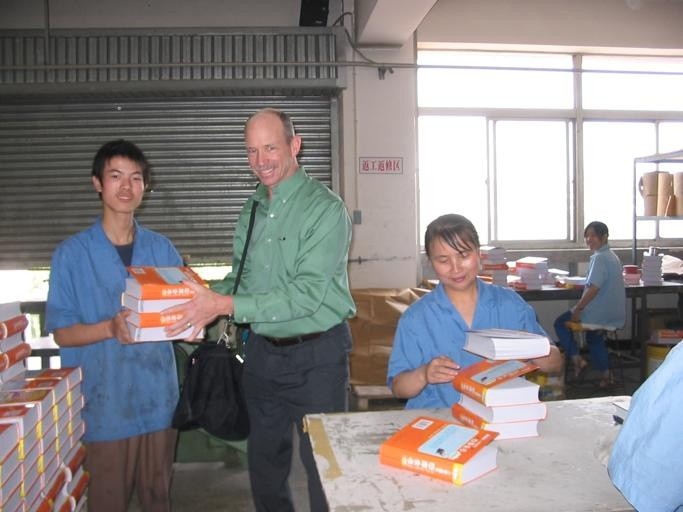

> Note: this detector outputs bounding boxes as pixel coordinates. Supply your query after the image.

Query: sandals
[570,356,616,390]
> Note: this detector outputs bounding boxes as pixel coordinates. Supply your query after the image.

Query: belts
[261,334,320,349]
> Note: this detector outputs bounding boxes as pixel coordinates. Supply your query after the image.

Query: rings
[187,323,191,327]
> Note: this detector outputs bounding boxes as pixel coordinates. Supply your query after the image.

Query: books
[124,265,210,300]
[121,292,193,313]
[365,321,551,488]
[123,308,184,327]
[460,234,664,291]
[609,396,630,425]
[0,295,89,512]
[126,322,205,342]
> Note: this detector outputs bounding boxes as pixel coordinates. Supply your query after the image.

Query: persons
[388,216,563,415]
[45,140,186,512]
[550,222,626,368]
[609,338,683,512]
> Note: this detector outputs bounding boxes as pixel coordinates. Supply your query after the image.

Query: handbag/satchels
[169,337,249,442]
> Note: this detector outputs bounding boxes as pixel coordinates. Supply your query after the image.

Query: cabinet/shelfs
[633,149,683,363]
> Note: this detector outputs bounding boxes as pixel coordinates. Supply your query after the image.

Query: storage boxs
[637,307,681,340]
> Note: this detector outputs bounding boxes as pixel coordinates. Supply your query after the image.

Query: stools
[564,321,630,396]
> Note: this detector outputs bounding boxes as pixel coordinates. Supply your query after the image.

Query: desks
[426,273,682,383]
[305,393,634,511]
